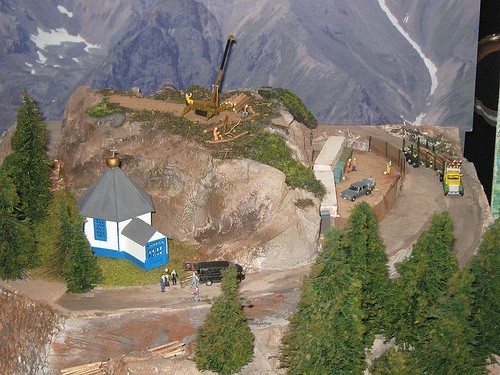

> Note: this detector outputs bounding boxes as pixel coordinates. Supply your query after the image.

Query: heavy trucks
[402,137,465,197]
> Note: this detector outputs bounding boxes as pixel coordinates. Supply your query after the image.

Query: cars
[339,178,375,202]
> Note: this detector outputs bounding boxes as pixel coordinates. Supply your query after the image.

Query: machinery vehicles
[179,34,235,121]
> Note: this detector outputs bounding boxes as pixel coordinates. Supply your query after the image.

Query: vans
[197,261,245,286]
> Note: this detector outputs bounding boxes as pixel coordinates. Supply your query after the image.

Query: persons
[345,157,393,196]
[159,268,201,302]
